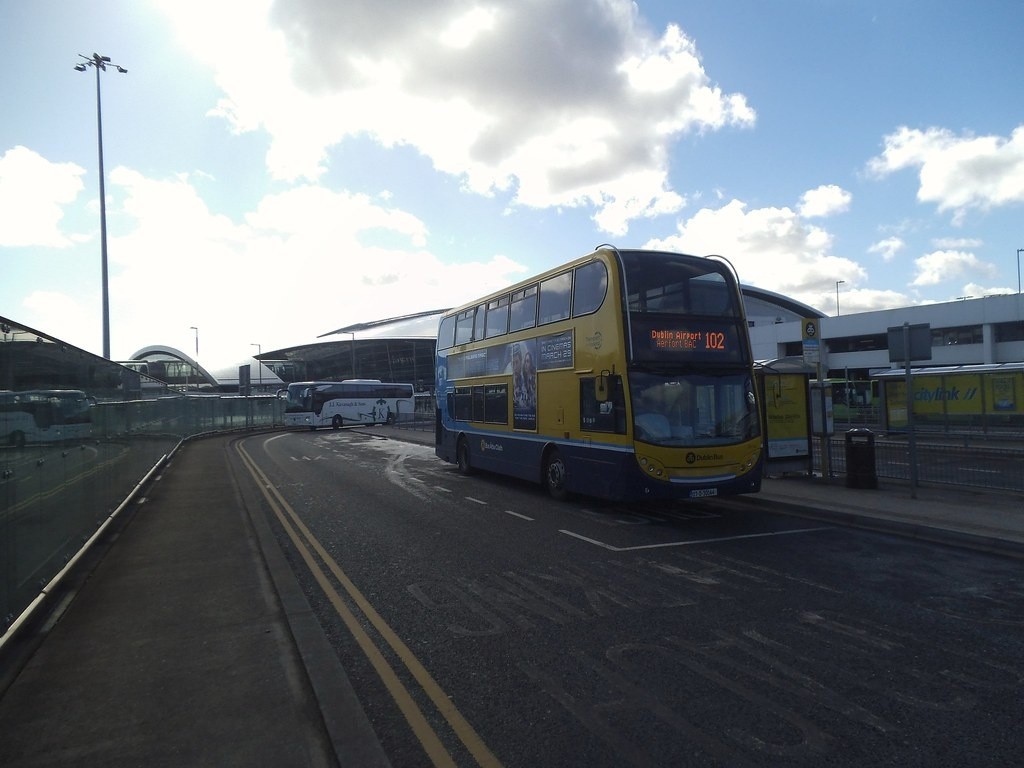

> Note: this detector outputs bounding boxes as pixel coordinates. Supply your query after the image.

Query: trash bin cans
[844,428,878,489]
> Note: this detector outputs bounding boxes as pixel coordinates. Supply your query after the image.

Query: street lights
[343,331,356,380]
[190,326,199,391]
[251,343,262,389]
[836,280,845,316]
[1017,248,1024,295]
[73,50,129,362]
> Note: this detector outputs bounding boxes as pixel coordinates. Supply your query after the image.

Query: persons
[509,343,537,408]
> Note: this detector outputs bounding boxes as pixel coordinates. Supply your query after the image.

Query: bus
[809,378,860,422]
[274,379,417,432]
[870,380,882,421]
[0,389,98,449]
[432,242,784,511]
